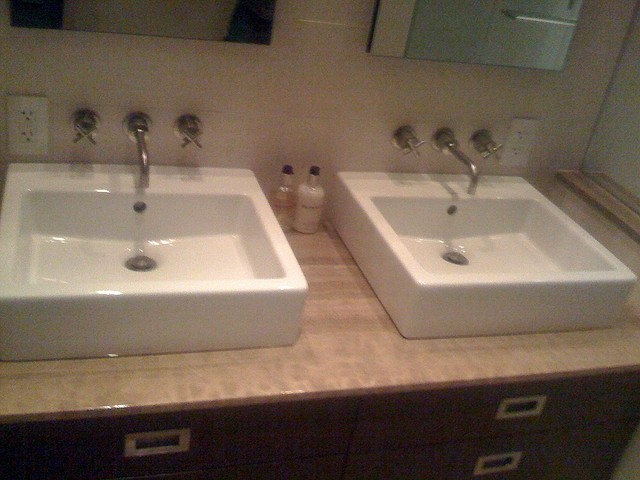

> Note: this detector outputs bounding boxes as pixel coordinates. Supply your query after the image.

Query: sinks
[10,190,286,285]
[370,194,617,277]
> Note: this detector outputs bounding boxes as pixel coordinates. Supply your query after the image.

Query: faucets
[432,128,479,196]
[121,111,153,187]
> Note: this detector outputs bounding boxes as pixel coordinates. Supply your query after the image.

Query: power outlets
[500,116,541,168]
[4,95,52,157]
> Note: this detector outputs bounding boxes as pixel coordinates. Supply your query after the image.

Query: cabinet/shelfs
[1,362,639,477]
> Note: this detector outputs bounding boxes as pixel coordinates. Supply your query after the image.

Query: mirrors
[8,1,275,45]
[368,2,586,73]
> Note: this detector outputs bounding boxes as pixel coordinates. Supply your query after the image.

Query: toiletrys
[270,164,295,232]
[294,165,326,234]
